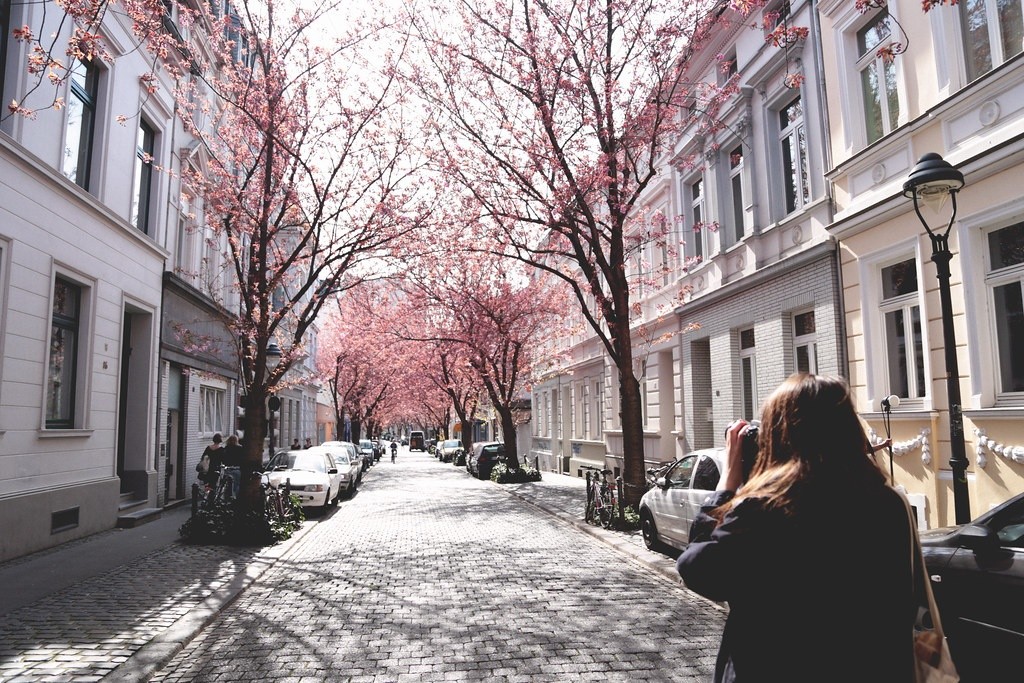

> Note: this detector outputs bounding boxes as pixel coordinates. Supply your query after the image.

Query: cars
[260,449,340,516]
[306,446,359,498]
[915,488,1024,683]
[381,433,409,446]
[638,445,727,552]
[466,442,504,480]
[320,440,387,482]
[433,439,464,462]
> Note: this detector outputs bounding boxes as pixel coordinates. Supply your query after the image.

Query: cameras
[724,421,761,463]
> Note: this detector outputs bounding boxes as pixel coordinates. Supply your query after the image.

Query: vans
[408,430,426,454]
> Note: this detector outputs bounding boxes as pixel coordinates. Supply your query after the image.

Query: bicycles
[392,447,397,463]
[579,456,679,529]
[204,464,301,532]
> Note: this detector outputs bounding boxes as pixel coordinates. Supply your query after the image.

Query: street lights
[900,152,972,526]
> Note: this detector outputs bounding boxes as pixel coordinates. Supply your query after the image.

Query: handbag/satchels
[888,485,960,683]
[196,456,209,472]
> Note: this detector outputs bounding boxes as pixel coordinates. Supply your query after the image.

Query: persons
[197,433,243,508]
[676,371,926,683]
[303,437,313,449]
[390,439,397,461]
[291,439,301,450]
